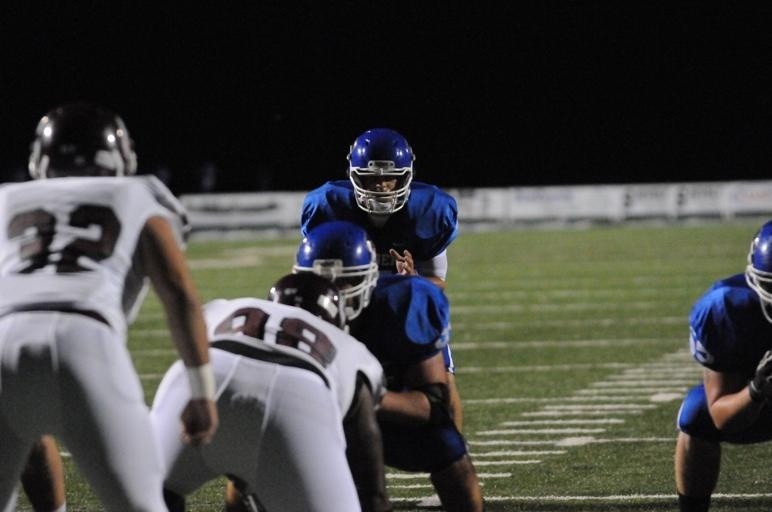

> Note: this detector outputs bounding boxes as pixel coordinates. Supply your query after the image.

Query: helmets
[28,99,138,178]
[347,128,416,177]
[748,220,772,280]
[267,220,379,330]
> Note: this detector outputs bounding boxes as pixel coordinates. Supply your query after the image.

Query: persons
[674,220,772,512]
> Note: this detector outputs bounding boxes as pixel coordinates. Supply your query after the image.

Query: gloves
[749,350,772,402]
